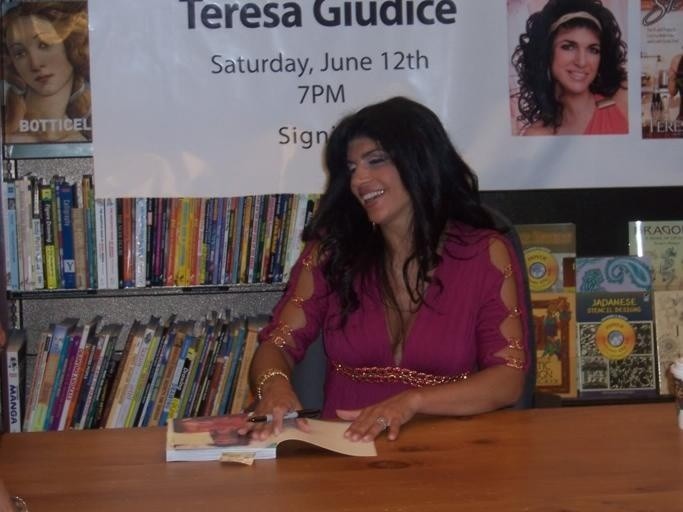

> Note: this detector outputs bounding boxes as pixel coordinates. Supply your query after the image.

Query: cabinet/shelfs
[0,145,315,431]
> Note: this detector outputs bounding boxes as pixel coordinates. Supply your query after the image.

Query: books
[95,194,320,290]
[1,307,272,435]
[509,213,682,409]
[164,413,382,463]
[1,172,98,291]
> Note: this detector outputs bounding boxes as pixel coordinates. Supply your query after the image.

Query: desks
[0,402,683,512]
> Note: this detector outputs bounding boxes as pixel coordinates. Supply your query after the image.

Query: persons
[665,50,681,128]
[507,1,630,136]
[173,417,254,448]
[0,1,95,149]
[233,95,536,445]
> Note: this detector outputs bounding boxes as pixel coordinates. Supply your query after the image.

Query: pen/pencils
[246,408,320,422]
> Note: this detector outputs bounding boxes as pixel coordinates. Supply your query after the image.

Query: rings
[374,416,388,430]
